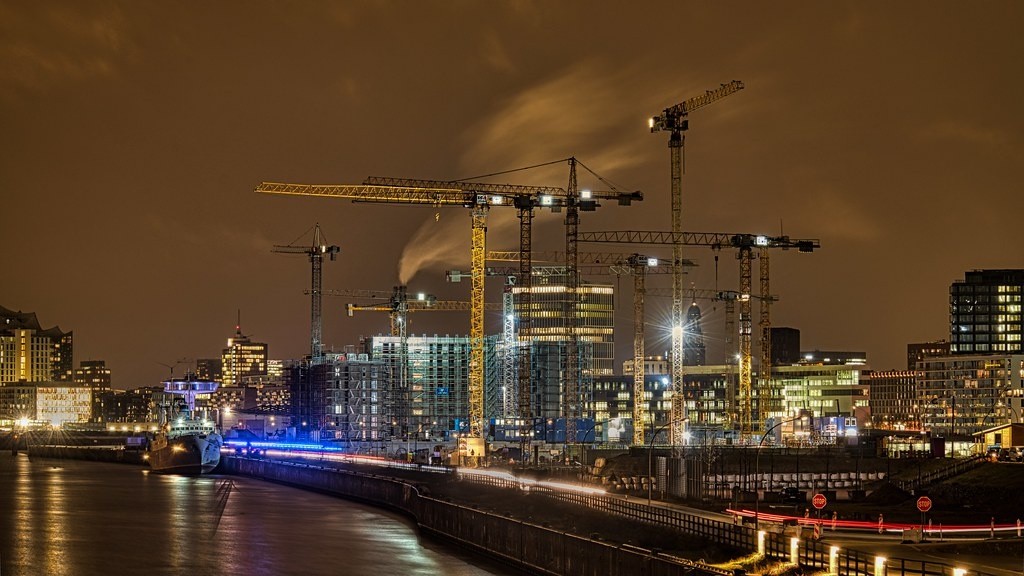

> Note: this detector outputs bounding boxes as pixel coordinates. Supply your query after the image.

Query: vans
[983,440,1024,462]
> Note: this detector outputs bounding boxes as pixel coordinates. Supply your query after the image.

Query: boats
[144,415,226,477]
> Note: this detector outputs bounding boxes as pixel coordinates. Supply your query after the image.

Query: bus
[427,456,440,467]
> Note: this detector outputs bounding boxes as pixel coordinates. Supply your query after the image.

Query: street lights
[923,395,943,455]
[755,413,816,531]
[979,383,1011,464]
[577,419,616,487]
[415,422,435,468]
[456,421,477,472]
[641,417,692,513]
[853,405,874,430]
[522,421,548,478]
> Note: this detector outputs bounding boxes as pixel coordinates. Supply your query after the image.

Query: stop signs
[917,496,932,511]
[812,494,826,509]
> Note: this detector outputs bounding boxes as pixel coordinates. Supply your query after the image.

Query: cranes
[251,75,822,454]
